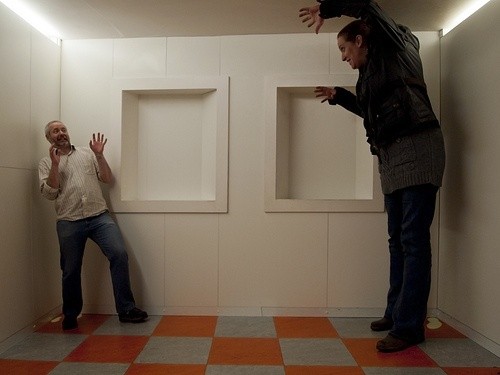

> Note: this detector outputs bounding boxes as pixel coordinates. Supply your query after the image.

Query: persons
[299,0,445,353]
[38,121,149,330]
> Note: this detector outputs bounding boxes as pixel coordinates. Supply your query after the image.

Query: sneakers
[377,333,425,352]
[62,315,77,329]
[118,307,148,322]
[371,317,393,331]
